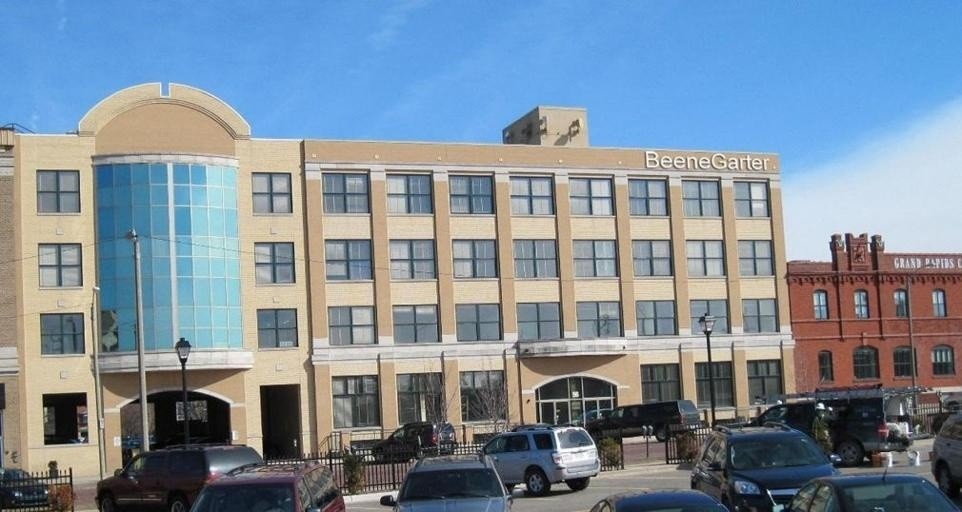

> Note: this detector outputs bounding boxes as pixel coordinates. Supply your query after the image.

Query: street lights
[86,281,105,485]
[699,313,720,425]
[906,263,935,415]
[173,337,193,442]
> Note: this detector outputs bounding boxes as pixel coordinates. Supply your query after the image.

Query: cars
[510,423,554,433]
[566,408,612,428]
[589,489,733,512]
[786,468,960,512]
[0,468,50,508]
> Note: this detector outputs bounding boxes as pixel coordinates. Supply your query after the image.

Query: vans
[587,400,701,444]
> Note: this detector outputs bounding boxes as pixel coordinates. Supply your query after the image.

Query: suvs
[484,422,601,498]
[745,387,915,469]
[191,460,348,512]
[927,409,962,497]
[94,443,263,512]
[372,419,457,465]
[689,418,840,511]
[379,449,523,512]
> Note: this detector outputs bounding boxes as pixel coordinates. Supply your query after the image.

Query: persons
[811,402,834,456]
